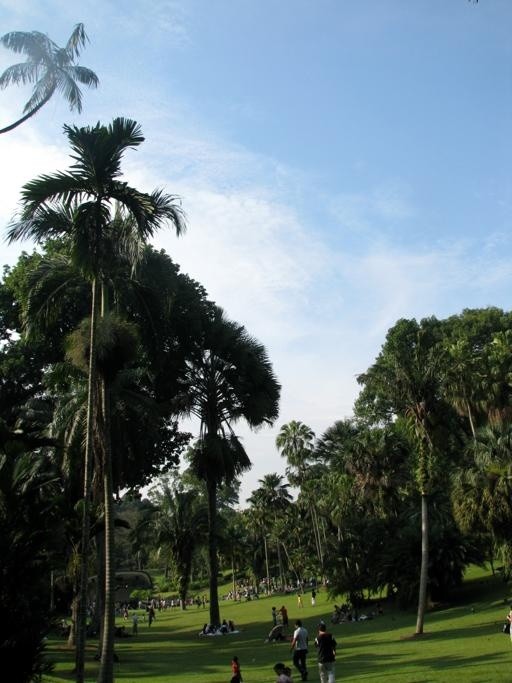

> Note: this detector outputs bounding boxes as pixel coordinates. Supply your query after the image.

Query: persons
[279,605,288,625]
[122,594,235,636]
[230,655,243,682]
[313,623,337,682]
[273,663,293,682]
[312,588,316,607]
[290,619,309,681]
[271,606,279,626]
[296,594,303,608]
[227,576,277,602]
